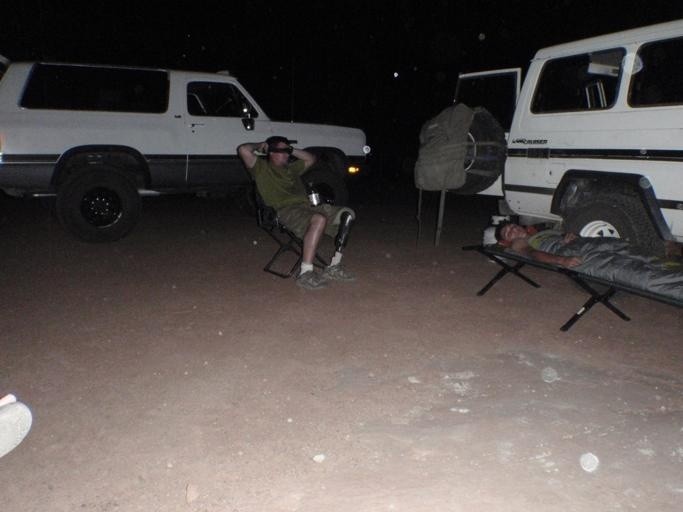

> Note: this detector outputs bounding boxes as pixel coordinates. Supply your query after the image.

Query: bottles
[308,182,321,206]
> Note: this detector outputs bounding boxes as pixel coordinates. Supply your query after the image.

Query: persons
[236,136,356,290]
[494,223,582,267]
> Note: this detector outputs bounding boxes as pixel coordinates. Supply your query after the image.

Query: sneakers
[321,263,358,281]
[296,270,327,289]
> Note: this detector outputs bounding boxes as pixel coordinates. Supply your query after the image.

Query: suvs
[445,20,683,257]
[0,59,366,242]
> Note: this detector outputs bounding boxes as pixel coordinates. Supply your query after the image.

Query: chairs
[239,154,336,280]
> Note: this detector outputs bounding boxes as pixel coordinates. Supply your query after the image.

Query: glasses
[268,145,293,154]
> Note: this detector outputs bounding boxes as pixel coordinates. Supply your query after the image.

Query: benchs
[462,241,683,333]
